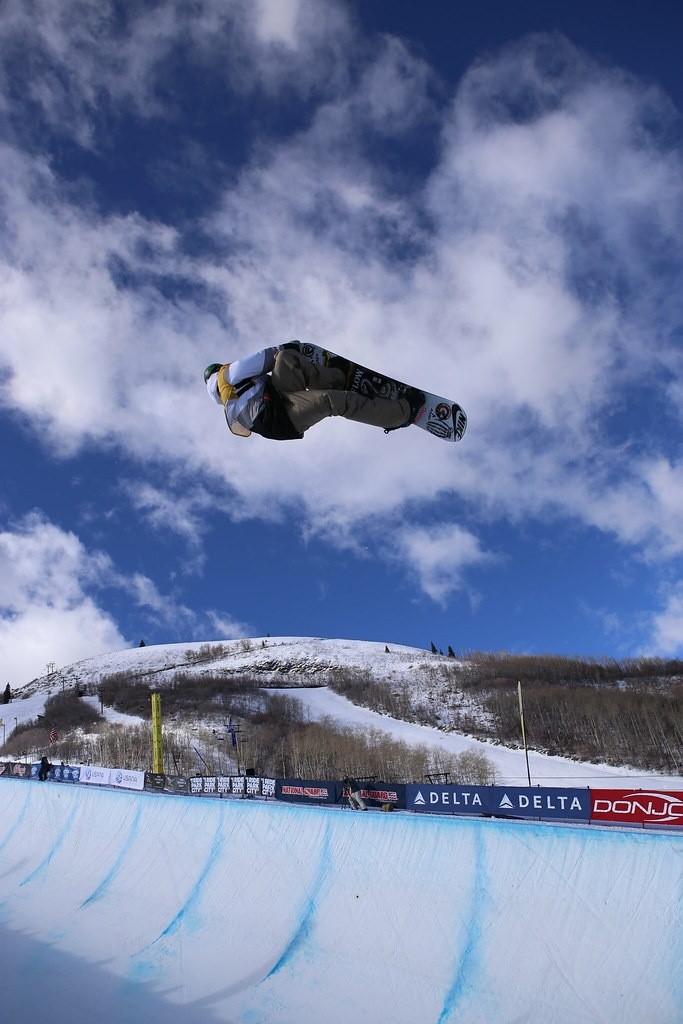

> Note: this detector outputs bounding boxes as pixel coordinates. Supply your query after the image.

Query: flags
[50,722,58,742]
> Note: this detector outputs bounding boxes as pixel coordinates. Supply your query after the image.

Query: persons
[343,775,367,811]
[203,339,426,440]
[39,756,48,781]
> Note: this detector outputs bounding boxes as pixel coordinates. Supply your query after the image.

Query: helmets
[204,364,222,384]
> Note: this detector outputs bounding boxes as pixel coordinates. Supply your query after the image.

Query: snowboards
[274,342,467,442]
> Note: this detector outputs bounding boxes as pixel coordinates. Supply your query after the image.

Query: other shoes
[331,357,348,390]
[404,388,426,425]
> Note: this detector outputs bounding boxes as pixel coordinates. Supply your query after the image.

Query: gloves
[284,343,301,353]
